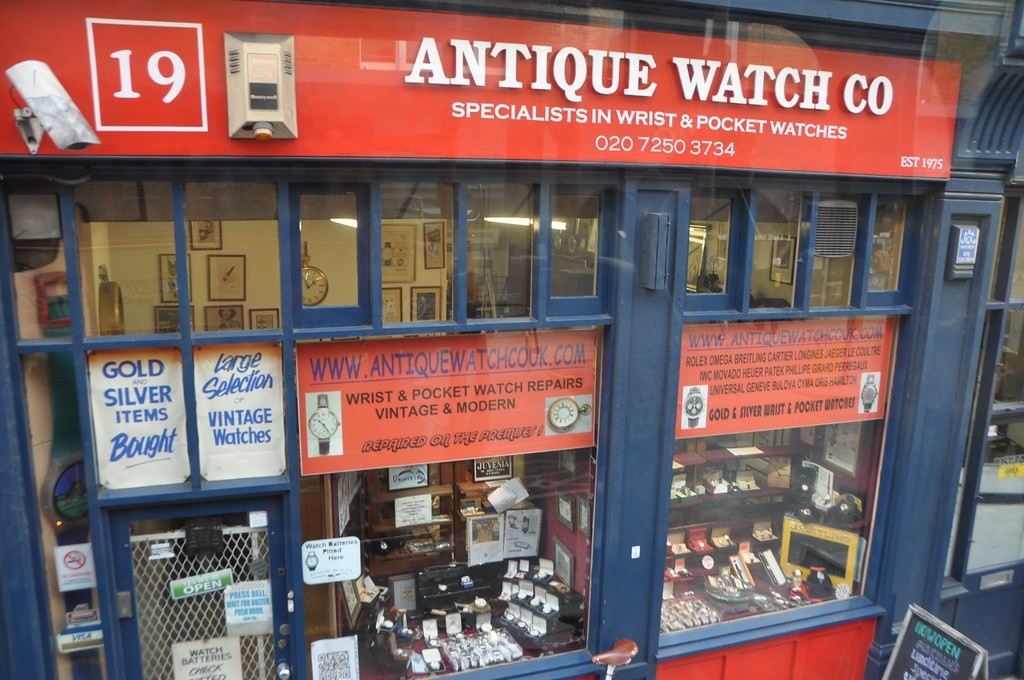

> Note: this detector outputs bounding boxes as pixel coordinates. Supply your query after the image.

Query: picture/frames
[577,493,592,540]
[159,254,191,303]
[553,534,575,590]
[337,580,362,630]
[423,222,445,269]
[208,254,246,301]
[382,223,417,283]
[769,235,797,285]
[382,287,402,322]
[556,487,576,532]
[155,305,194,333]
[190,220,222,250]
[205,305,244,331]
[558,449,580,483]
[388,573,417,611]
[249,308,280,330]
[410,286,442,321]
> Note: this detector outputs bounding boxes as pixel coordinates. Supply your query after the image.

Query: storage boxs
[687,528,714,556]
[388,633,410,660]
[667,529,693,559]
[476,612,493,634]
[444,612,466,640]
[498,557,562,636]
[710,525,738,552]
[421,619,441,649]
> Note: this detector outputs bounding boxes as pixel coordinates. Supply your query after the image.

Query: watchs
[681,475,751,496]
[760,528,773,539]
[676,535,733,553]
[522,516,529,534]
[308,395,340,454]
[668,568,693,578]
[816,571,825,584]
[717,569,809,611]
[747,552,755,563]
[363,576,523,673]
[861,374,877,413]
[683,387,705,428]
[660,591,720,634]
[499,571,571,639]
[305,551,319,571]
[800,483,849,519]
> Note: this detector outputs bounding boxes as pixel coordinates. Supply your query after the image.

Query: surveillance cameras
[6,59,102,150]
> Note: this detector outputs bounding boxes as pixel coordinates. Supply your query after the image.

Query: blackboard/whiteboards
[881,604,985,680]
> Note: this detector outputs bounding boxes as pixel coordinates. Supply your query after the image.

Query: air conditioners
[444,227,500,249]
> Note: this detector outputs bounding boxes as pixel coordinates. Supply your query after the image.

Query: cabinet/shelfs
[659,419,889,634]
[354,454,538,576]
[660,419,888,634]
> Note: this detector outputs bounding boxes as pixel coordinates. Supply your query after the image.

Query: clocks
[301,255,328,306]
